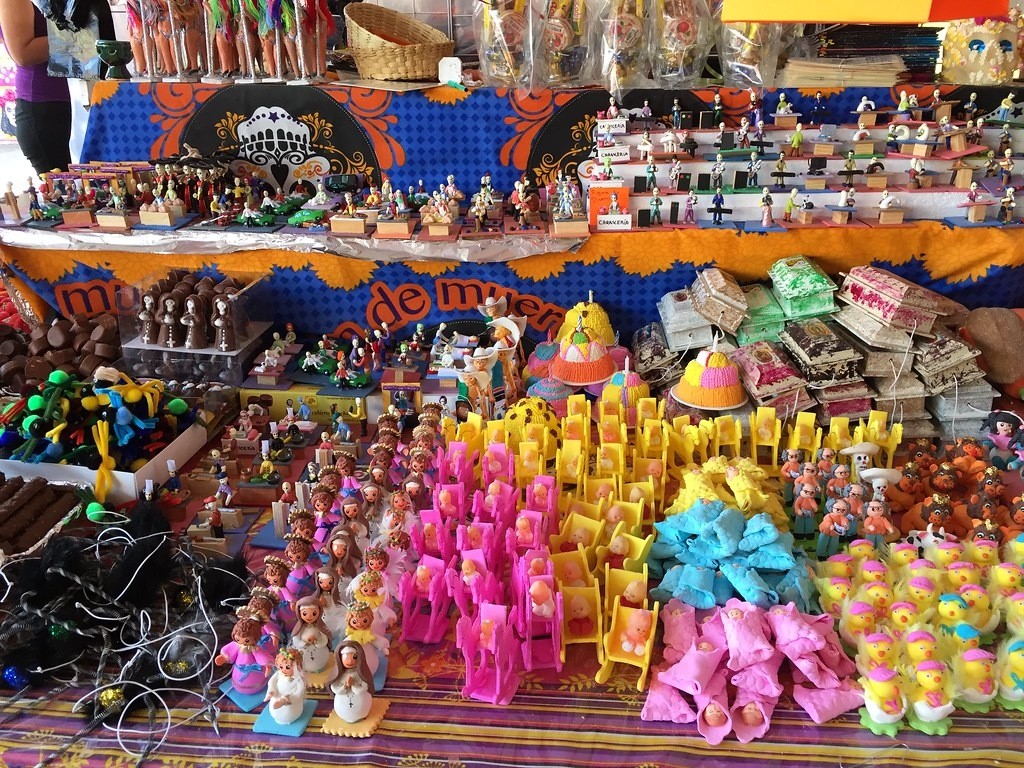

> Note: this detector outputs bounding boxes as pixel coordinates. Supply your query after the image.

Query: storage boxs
[364,380,468,424]
[0,378,207,510]
[240,383,365,426]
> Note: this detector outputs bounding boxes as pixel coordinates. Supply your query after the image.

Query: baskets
[343,2,453,82]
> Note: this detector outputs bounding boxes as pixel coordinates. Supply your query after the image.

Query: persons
[126,0,334,79]
[136,267,527,415]
[0,0,116,180]
[593,88,1018,223]
[4,154,580,234]
[980,407,1024,480]
[139,391,903,740]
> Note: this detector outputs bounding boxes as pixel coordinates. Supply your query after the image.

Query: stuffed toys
[822,438,1024,768]
